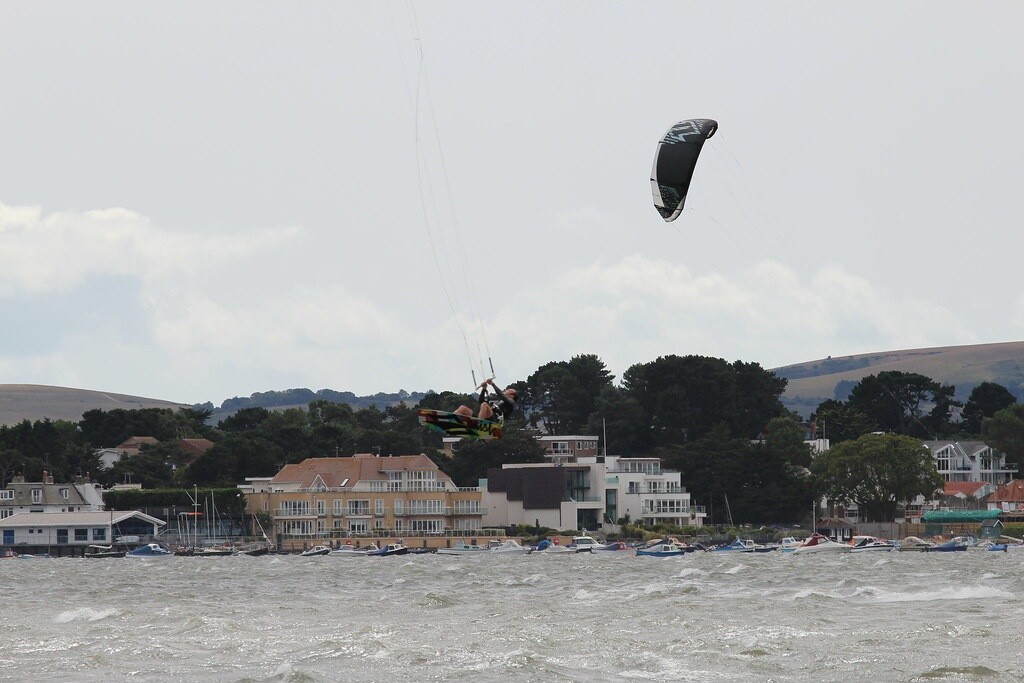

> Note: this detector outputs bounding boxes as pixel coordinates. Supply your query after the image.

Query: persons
[451,378,518,426]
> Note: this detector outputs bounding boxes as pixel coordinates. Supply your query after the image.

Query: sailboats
[173,485,272,555]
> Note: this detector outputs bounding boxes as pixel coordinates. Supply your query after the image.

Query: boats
[362,543,388,556]
[84,545,124,557]
[570,536,605,551]
[408,548,431,554]
[436,542,493,555]
[388,544,407,555]
[328,544,366,557]
[299,545,330,556]
[531,543,577,554]
[635,535,1007,557]
[491,540,531,555]
[126,543,174,557]
[604,541,625,550]
